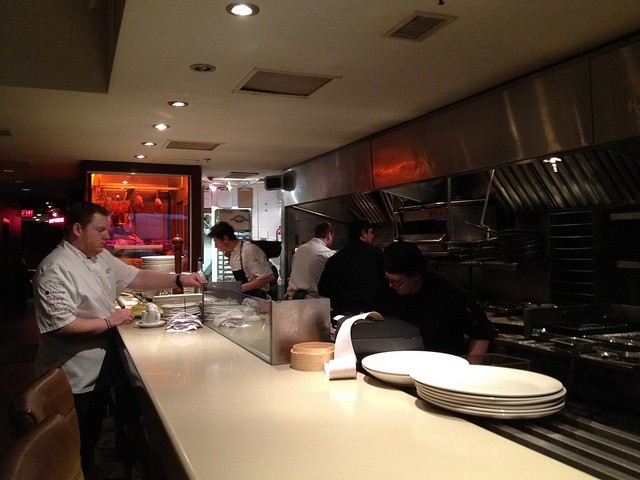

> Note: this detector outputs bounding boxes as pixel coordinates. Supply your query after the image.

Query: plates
[135,320,165,328]
[206,299,246,325]
[160,301,203,327]
[141,256,175,274]
[361,351,469,387]
[409,365,566,418]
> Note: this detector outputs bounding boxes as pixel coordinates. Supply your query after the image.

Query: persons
[286,222,338,300]
[317,220,387,329]
[375,241,495,365]
[208,221,275,300]
[33,201,206,480]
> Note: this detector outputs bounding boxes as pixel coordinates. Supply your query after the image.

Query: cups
[140,311,161,323]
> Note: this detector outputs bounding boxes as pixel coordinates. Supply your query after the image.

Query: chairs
[0,413,86,480]
[9,368,81,448]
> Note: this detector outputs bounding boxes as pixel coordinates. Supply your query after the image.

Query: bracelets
[176,274,183,290]
[104,317,111,330]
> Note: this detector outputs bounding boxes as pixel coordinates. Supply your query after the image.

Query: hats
[382,240,424,277]
[348,218,373,230]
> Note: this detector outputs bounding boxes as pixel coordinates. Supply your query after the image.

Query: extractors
[388,198,500,263]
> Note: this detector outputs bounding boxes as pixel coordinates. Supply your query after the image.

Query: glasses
[384,276,410,288]
